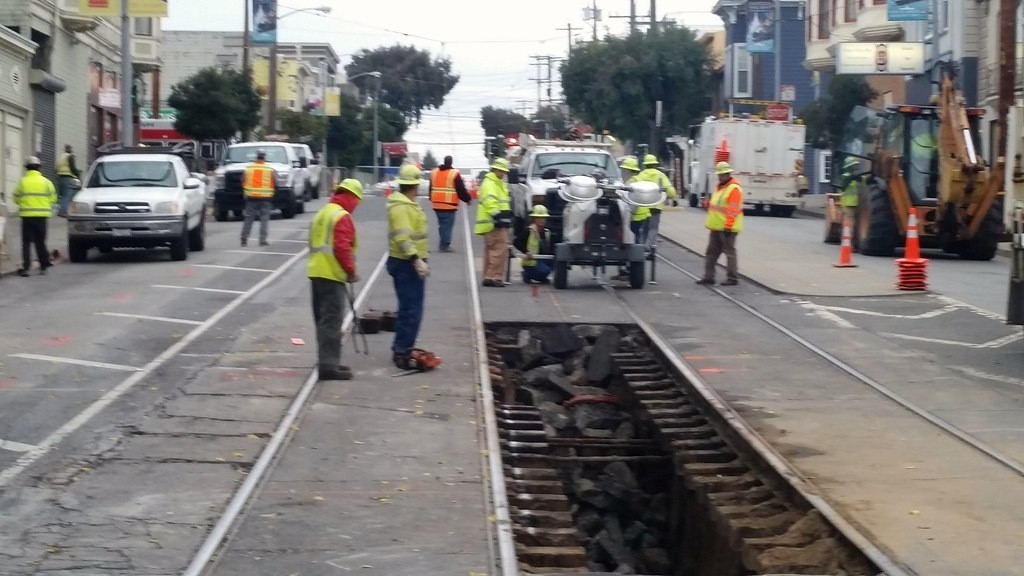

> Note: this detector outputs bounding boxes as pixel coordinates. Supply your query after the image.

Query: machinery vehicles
[823,57,1007,261]
[1002,85,1024,325]
[683,99,810,218]
[537,172,659,290]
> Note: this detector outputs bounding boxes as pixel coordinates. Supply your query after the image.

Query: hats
[444,156,452,164]
[841,156,862,168]
[256,149,265,157]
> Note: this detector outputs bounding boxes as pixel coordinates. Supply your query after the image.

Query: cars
[414,169,434,196]
[460,174,478,198]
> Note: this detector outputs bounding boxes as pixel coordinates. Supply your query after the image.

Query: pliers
[340,280,371,356]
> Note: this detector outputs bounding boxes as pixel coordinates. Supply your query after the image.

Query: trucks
[505,132,626,261]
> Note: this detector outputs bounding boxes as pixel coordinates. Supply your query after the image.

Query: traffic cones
[834,217,861,268]
[714,138,731,167]
[894,209,931,291]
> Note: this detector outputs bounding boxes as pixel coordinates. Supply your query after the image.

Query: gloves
[412,259,432,280]
[466,199,474,207]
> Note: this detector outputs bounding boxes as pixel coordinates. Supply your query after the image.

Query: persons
[429,156,470,252]
[386,165,430,360]
[307,179,364,379]
[838,156,868,252]
[13,156,58,275]
[513,205,554,282]
[476,157,512,286]
[240,149,277,246]
[55,145,80,218]
[911,131,939,160]
[611,158,652,280]
[696,162,742,286]
[640,155,677,259]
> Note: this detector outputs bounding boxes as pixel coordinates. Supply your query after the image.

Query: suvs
[287,143,323,202]
[203,141,311,220]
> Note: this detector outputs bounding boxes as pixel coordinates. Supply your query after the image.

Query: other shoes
[319,371,352,380]
[239,236,247,246]
[258,241,270,246]
[39,268,47,276]
[481,278,504,287]
[438,246,454,252]
[332,365,351,372]
[719,280,737,286]
[695,279,715,285]
[56,210,69,217]
[17,269,30,276]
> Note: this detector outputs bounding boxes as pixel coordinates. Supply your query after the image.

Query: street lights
[267,6,331,126]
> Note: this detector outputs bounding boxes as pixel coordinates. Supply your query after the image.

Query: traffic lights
[492,143,500,157]
[484,144,488,158]
[531,120,544,139]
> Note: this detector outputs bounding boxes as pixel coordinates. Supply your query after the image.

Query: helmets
[397,165,421,185]
[619,158,640,172]
[642,154,659,165]
[601,129,611,135]
[713,162,734,175]
[488,158,510,173]
[331,178,364,200]
[527,205,550,217]
[22,155,42,167]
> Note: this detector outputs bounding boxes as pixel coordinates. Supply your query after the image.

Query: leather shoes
[536,278,550,284]
[524,276,541,285]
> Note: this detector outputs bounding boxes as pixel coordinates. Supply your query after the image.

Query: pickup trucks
[64,153,208,262]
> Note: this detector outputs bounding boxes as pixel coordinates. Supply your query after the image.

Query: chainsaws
[390,348,441,378]
